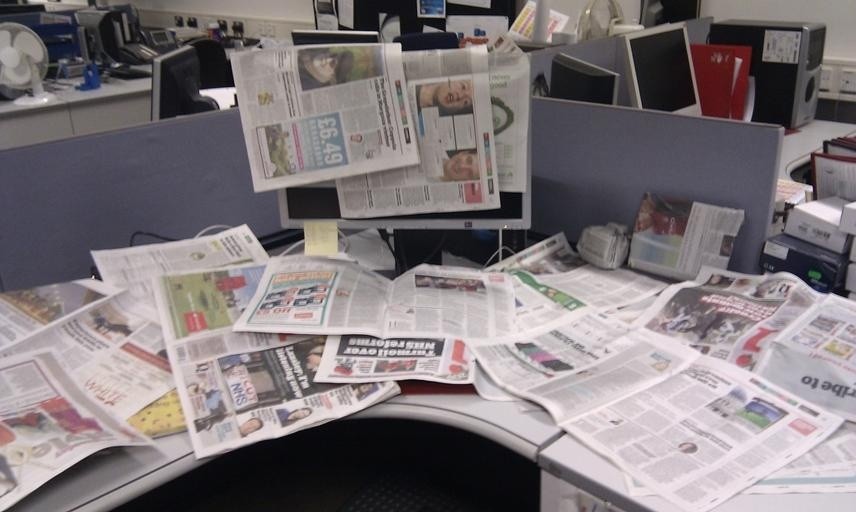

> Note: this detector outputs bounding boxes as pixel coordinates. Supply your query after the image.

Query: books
[766,133,856,239]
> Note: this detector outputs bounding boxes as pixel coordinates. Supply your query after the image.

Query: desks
[1,233,856,511]
[0,64,159,153]
[776,119,856,204]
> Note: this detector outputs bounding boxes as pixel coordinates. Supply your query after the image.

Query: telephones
[139,26,178,55]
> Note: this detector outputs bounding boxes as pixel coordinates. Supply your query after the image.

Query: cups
[206,21,221,41]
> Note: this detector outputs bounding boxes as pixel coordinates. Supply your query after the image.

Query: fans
[0,21,51,111]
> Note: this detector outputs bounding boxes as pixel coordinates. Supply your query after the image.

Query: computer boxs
[710,18,826,129]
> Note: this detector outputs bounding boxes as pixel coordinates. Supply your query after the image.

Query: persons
[441,148,479,180]
[236,418,262,438]
[490,95,514,137]
[276,406,311,427]
[352,383,373,399]
[414,80,472,110]
[265,125,289,151]
[298,49,353,91]
[632,191,695,239]
[350,134,363,143]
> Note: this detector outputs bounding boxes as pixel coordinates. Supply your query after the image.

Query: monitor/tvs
[550,53,620,105]
[291,31,382,46]
[150,44,219,122]
[619,21,703,120]
[278,51,533,264]
